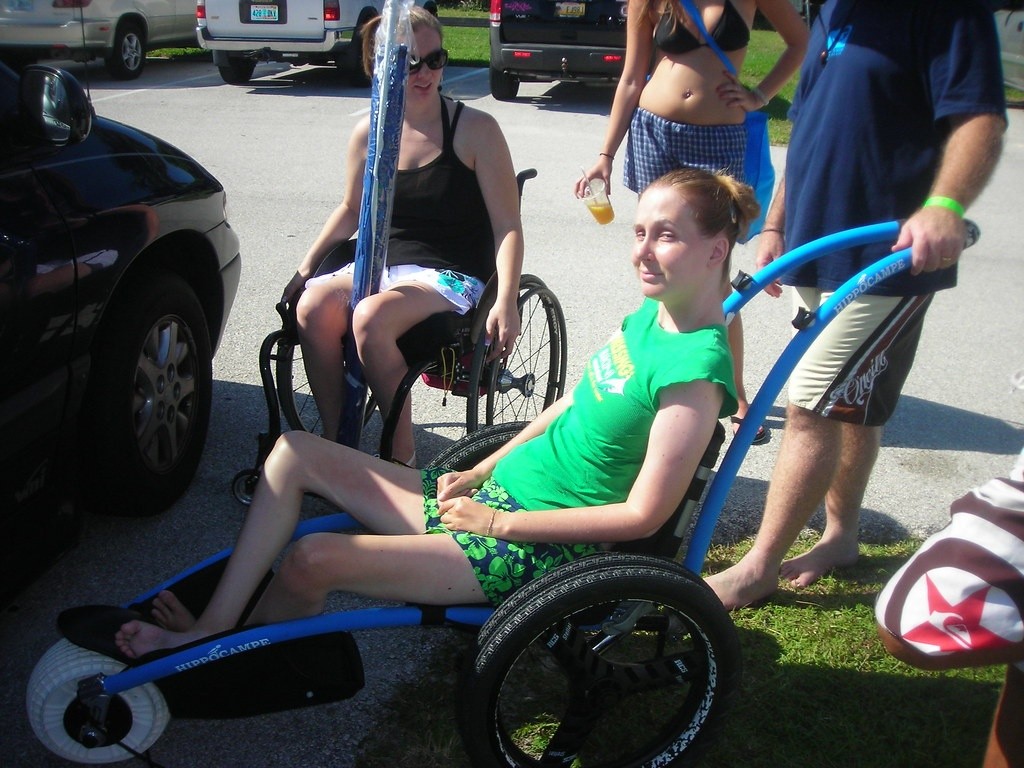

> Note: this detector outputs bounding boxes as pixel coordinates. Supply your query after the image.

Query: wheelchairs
[231,172,569,508]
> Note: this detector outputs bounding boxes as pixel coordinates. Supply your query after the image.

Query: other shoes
[374,451,416,470]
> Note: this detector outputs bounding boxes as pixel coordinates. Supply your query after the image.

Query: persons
[574,0,808,443]
[703,0,1009,612]
[115,169,760,661]
[874,445,1024,768]
[281,6,523,470]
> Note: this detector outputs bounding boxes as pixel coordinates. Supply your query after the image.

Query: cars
[194,0,437,87]
[485,0,653,102]
[1,0,200,82]
[1,62,242,607]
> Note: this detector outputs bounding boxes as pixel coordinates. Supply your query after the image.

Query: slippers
[732,414,767,445]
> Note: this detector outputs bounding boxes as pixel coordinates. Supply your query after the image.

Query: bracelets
[486,509,497,537]
[924,197,964,219]
[761,229,785,235]
[752,87,769,106]
[599,153,614,159]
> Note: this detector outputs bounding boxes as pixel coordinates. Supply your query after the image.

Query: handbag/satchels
[735,111,775,244]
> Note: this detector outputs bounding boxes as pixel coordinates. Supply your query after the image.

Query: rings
[941,257,952,265]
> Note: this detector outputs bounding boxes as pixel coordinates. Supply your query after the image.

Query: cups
[578,178,615,225]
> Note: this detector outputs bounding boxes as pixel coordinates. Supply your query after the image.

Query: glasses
[408,49,448,75]
